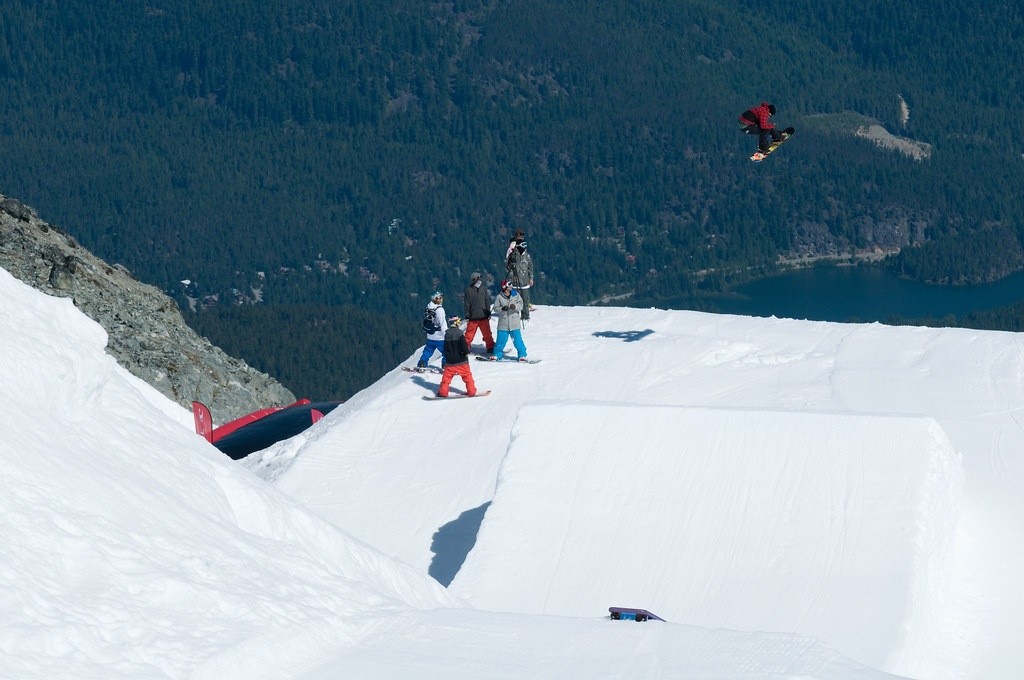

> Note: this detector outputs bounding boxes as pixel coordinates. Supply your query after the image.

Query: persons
[435,315,477,397]
[464,272,496,353]
[737,102,787,155]
[505,239,534,319]
[490,280,528,361]
[417,290,448,371]
[506,231,525,259]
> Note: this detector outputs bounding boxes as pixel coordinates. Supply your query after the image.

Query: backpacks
[422,306,443,335]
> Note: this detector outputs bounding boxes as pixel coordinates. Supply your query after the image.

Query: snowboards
[401,364,458,376]
[749,126,796,163]
[421,390,491,402]
[476,356,542,365]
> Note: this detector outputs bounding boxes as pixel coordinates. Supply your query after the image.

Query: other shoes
[419,361,426,367]
[490,356,502,362]
[519,357,525,362]
[442,363,445,369]
[437,393,444,397]
[487,348,493,353]
[758,148,770,155]
[771,135,787,141]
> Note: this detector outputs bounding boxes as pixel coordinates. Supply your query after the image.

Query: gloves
[502,306,508,311]
[510,304,515,310]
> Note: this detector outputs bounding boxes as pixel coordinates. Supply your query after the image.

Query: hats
[501,279,509,292]
[470,272,482,286]
[768,105,776,114]
[515,229,524,235]
[516,239,527,250]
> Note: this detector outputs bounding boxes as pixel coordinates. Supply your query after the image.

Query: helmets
[430,290,444,301]
[448,314,459,322]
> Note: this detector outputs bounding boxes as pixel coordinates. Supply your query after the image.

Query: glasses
[519,242,527,248]
[457,318,462,324]
[520,232,525,236]
[478,275,483,281]
[506,281,513,288]
